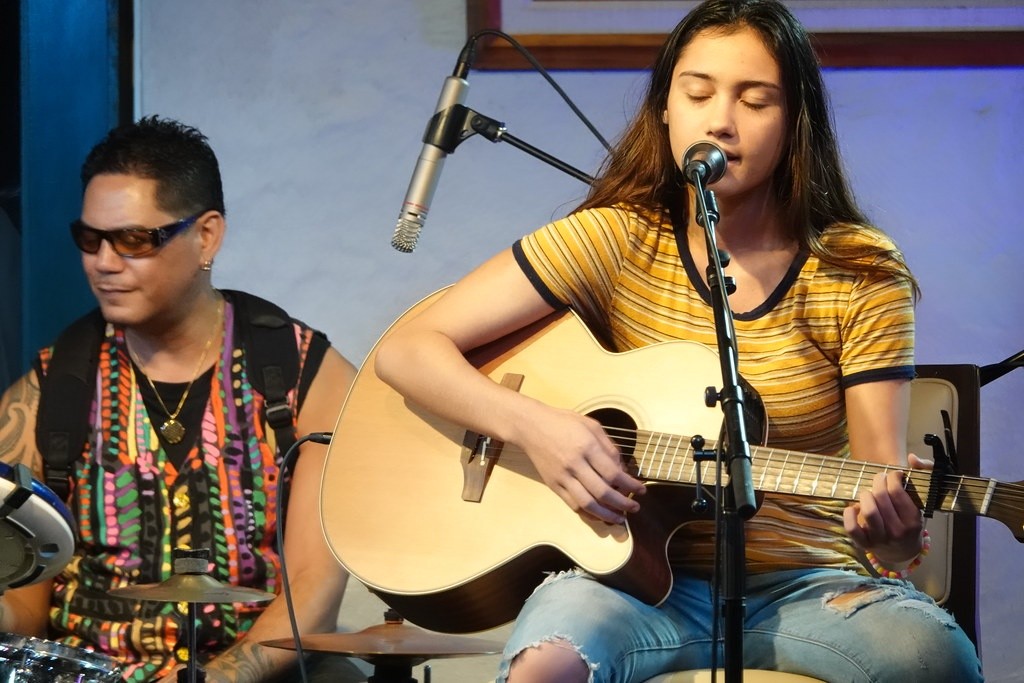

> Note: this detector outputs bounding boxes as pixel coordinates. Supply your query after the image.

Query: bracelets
[865,530,931,580]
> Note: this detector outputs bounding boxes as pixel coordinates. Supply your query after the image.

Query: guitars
[319,281,1024,634]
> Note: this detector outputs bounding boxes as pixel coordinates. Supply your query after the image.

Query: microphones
[683,139,728,186]
[389,36,480,252]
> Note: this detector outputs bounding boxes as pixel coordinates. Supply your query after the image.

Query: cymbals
[258,622,509,666]
[105,575,277,604]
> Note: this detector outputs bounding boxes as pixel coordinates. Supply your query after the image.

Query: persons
[0,114,362,683]
[375,1,982,683]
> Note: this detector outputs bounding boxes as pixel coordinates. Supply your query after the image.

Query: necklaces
[124,288,227,443]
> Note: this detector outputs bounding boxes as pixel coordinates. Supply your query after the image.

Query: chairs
[642,360,985,683]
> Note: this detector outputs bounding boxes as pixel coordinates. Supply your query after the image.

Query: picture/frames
[459,0,1024,74]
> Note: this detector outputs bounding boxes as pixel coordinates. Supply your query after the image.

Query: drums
[0,632,123,682]
[0,462,76,599]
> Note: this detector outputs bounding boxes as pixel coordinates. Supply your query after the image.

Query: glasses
[70,205,217,259]
